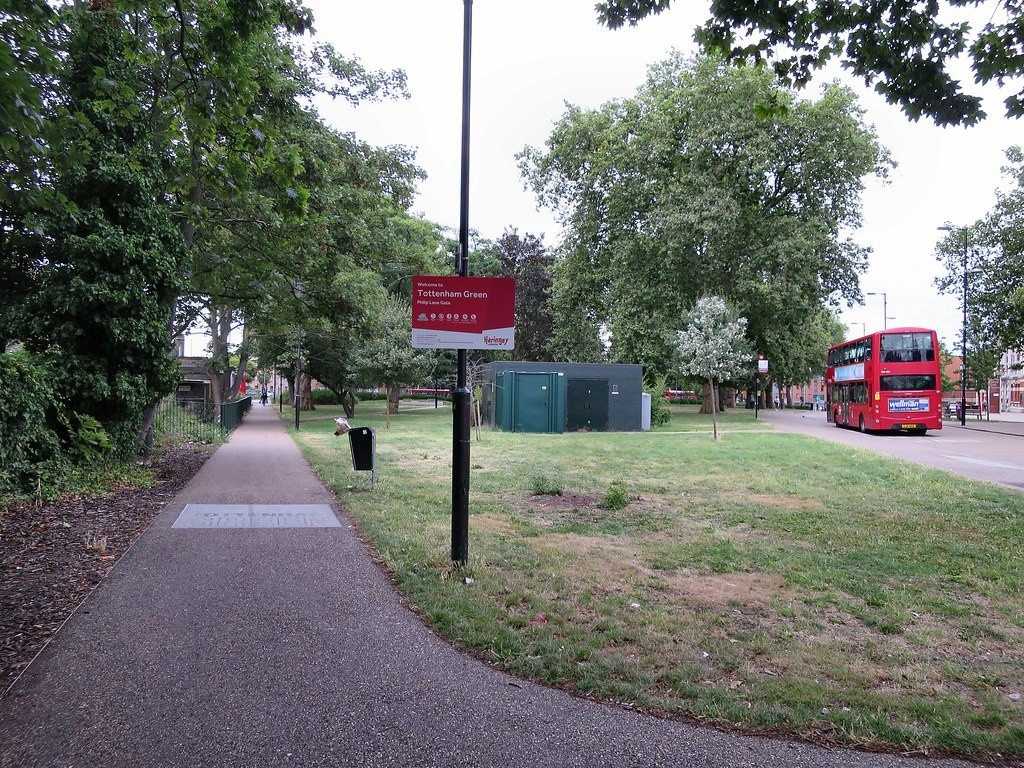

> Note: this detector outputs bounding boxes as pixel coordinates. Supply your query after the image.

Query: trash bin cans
[956,401,962,421]
[349,426,375,471]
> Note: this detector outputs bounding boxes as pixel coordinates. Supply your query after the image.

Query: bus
[662,389,698,400]
[826,327,942,436]
[405,389,449,397]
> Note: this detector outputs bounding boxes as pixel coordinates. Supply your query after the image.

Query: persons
[774,396,779,411]
[255,384,268,403]
[780,394,784,410]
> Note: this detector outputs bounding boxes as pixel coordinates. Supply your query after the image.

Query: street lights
[850,322,867,336]
[936,226,968,426]
[866,292,886,329]
